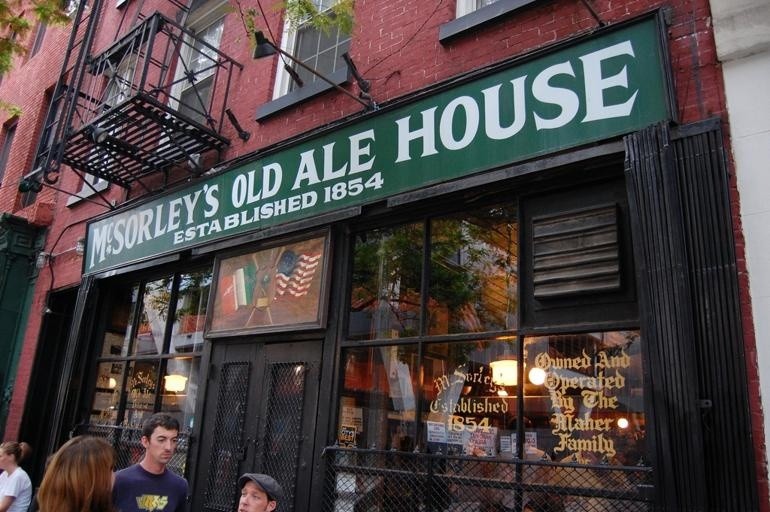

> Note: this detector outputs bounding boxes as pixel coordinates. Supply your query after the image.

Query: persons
[389,416,634,511]
[0,441,33,512]
[237,472,284,512]
[36,435,119,512]
[112,412,190,512]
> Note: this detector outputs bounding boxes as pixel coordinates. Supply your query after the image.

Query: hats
[238,472,285,501]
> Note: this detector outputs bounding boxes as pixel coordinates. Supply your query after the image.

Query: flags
[220,250,322,315]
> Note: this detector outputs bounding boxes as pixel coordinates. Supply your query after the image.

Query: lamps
[35,237,87,270]
[11,176,110,208]
[248,31,372,110]
[85,118,182,187]
